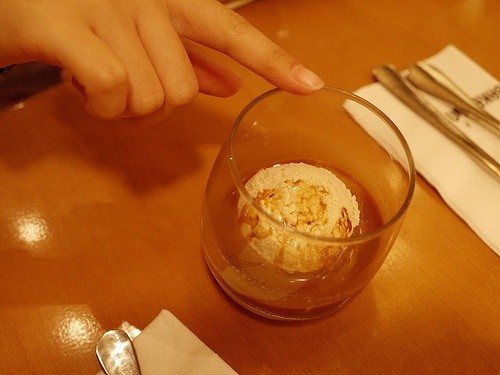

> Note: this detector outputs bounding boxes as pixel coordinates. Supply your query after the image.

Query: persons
[0,0,326,122]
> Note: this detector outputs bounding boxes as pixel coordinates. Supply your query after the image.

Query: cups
[199,85,416,321]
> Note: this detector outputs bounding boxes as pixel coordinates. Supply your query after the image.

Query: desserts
[237,163,360,273]
